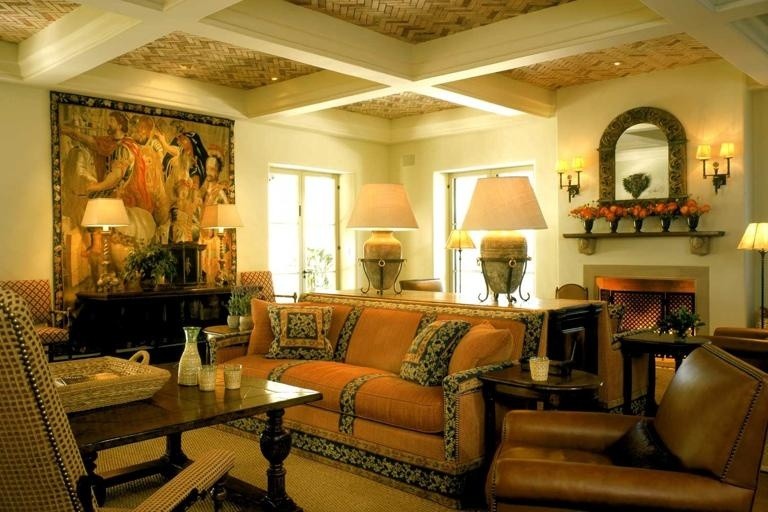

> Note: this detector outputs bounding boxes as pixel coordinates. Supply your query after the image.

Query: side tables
[202,326,248,365]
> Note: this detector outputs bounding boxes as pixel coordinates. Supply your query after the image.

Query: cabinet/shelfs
[75,287,231,365]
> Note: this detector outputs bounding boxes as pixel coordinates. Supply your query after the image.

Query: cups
[529,356,549,382]
[197,364,217,391]
[223,363,243,391]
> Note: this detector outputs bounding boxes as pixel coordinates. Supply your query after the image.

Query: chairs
[555,283,589,300]
[0,278,73,362]
[0,290,234,512]
[241,271,297,303]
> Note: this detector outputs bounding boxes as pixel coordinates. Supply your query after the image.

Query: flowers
[567,199,711,222]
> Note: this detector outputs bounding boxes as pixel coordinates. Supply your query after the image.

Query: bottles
[177,326,202,388]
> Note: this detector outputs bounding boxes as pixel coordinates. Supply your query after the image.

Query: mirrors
[595,106,689,217]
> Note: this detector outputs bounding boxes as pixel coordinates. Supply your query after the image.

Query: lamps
[201,203,244,286]
[80,198,130,291]
[346,176,547,302]
[695,143,735,195]
[553,157,584,203]
[736,223,768,329]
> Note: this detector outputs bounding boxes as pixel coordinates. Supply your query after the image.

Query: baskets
[48,350,171,413]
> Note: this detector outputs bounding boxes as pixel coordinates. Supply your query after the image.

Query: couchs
[598,301,651,408]
[209,295,546,510]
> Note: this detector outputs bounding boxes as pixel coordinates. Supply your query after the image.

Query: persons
[59,105,231,284]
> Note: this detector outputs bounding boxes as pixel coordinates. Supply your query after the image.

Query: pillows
[399,319,514,387]
[246,298,335,361]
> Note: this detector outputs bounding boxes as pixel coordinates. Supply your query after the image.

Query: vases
[583,216,700,234]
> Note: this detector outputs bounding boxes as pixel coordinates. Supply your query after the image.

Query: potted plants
[226,287,258,330]
[656,304,701,342]
[124,235,176,290]
